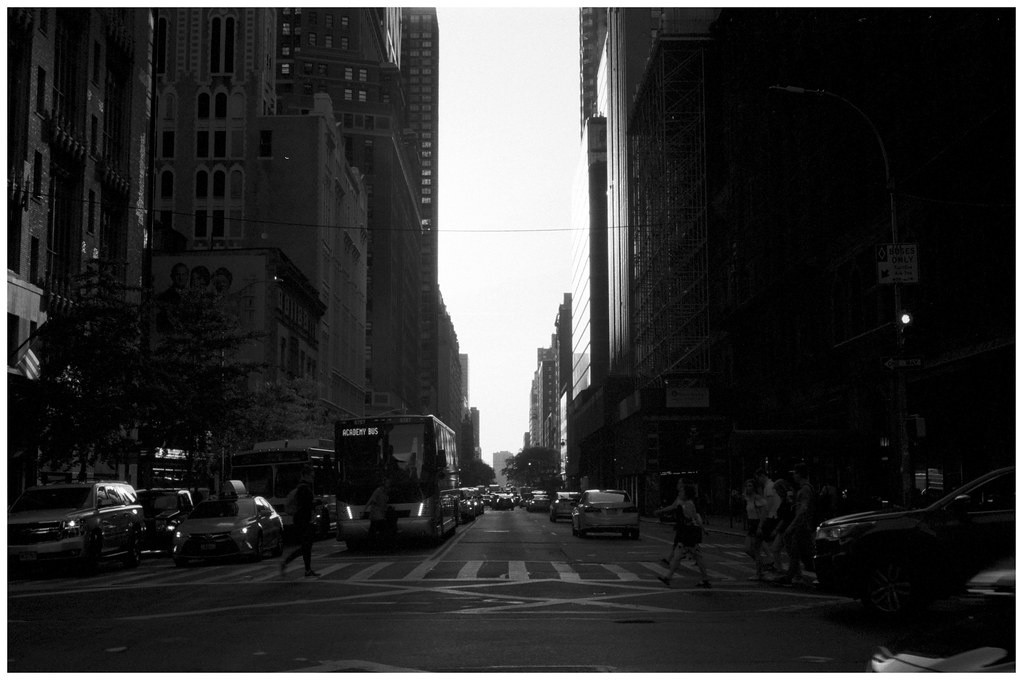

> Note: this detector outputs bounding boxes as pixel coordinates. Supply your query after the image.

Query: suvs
[814,465,1015,617]
[7,480,144,574]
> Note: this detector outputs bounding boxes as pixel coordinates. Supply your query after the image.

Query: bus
[228,438,336,541]
[333,414,460,548]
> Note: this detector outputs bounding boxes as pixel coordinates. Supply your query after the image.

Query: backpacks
[803,483,825,526]
[283,483,309,515]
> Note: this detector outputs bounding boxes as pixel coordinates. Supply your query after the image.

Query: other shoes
[748,572,803,587]
[305,570,321,578]
[661,558,670,565]
[812,580,819,585]
[694,580,711,586]
[657,575,670,585]
[280,562,287,572]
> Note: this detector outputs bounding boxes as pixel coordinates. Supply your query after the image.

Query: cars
[572,490,639,538]
[549,492,582,522]
[459,483,551,521]
[136,488,194,555]
[953,557,1015,627]
[173,480,284,562]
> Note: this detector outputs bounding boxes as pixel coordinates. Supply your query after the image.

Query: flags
[16,338,52,385]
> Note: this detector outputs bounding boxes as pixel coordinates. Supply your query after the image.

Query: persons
[359,477,395,556]
[281,464,328,578]
[741,463,846,587]
[656,477,703,567]
[154,262,243,331]
[657,484,712,590]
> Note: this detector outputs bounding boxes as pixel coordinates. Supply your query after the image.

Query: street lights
[768,84,915,505]
